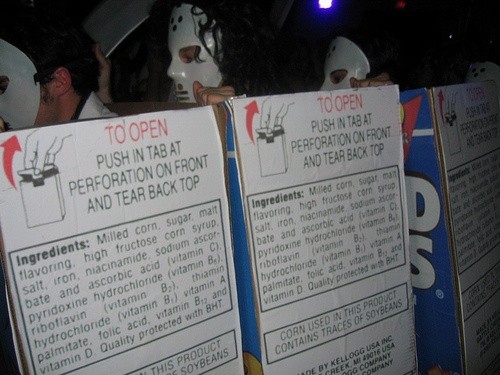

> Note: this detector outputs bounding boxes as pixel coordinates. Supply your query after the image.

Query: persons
[318,32,395,94]
[160,0,286,109]
[0,6,121,135]
